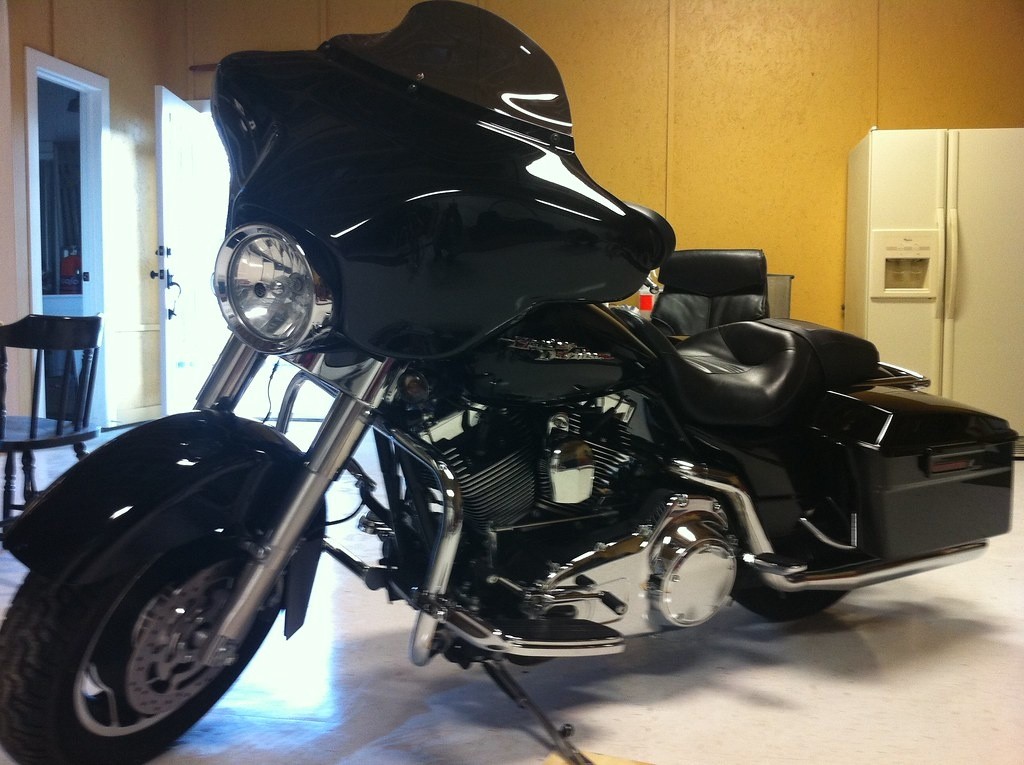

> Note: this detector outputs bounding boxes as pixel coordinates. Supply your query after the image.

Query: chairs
[0,313,107,539]
[651,248,772,338]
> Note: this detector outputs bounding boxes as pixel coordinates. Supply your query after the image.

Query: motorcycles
[0,1,1018,764]
